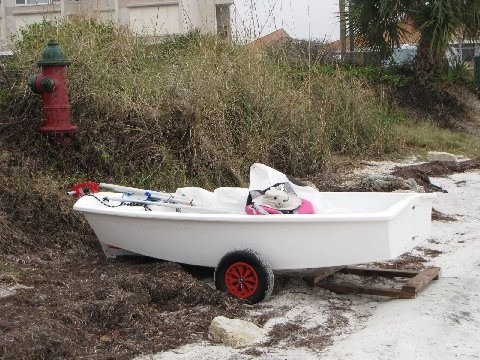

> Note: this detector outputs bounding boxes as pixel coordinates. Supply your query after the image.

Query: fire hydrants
[28,37,78,147]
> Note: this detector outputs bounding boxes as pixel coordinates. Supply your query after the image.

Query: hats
[253,189,302,211]
